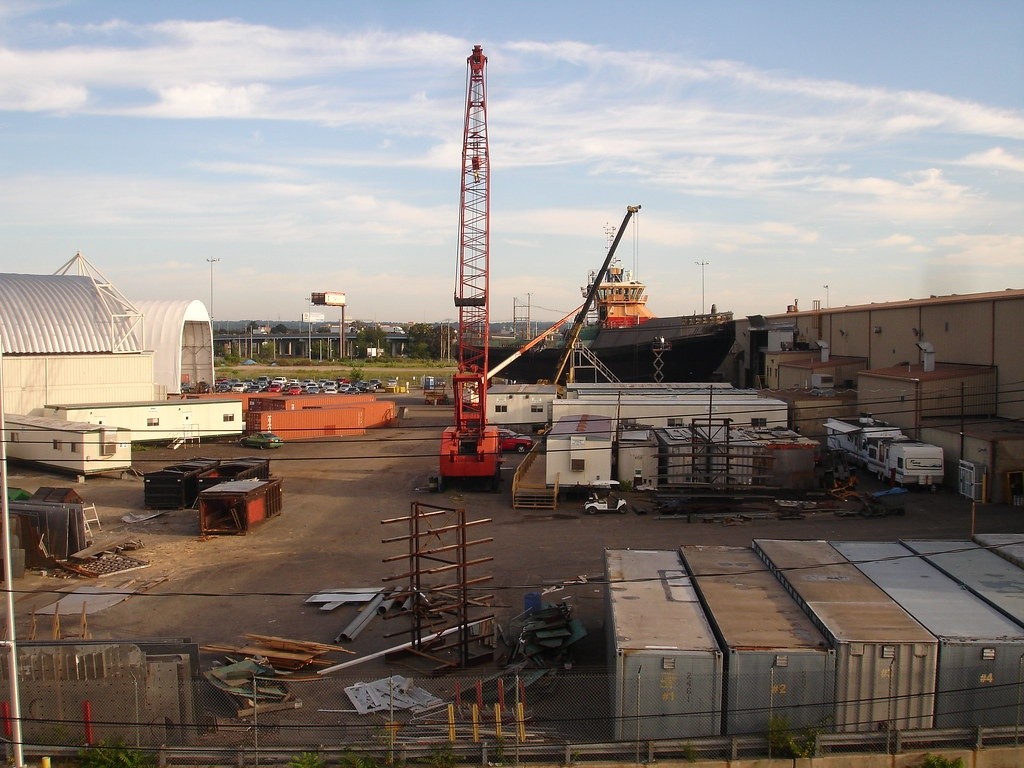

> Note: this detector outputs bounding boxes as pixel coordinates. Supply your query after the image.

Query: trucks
[423,391,449,405]
[827,414,948,492]
[253,329,267,335]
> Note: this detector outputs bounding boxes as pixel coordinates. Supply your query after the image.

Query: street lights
[305,296,312,362]
[694,260,710,314]
[206,256,222,333]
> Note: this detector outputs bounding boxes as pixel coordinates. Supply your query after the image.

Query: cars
[307,386,320,394]
[180,381,210,393]
[289,386,303,396]
[242,360,258,366]
[344,387,360,395]
[215,375,382,395]
[435,378,446,388]
[325,388,338,395]
[499,428,535,453]
[387,380,398,387]
[240,431,284,450]
[268,383,281,393]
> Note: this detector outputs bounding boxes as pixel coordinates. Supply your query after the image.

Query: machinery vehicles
[433,42,511,493]
[455,299,588,415]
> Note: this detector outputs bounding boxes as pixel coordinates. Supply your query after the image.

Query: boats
[459,224,737,382]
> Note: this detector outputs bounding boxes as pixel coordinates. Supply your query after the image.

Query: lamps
[911,327,924,336]
[977,447,987,452]
[839,329,848,336]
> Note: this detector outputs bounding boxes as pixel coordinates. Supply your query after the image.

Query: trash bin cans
[524,592,541,610]
